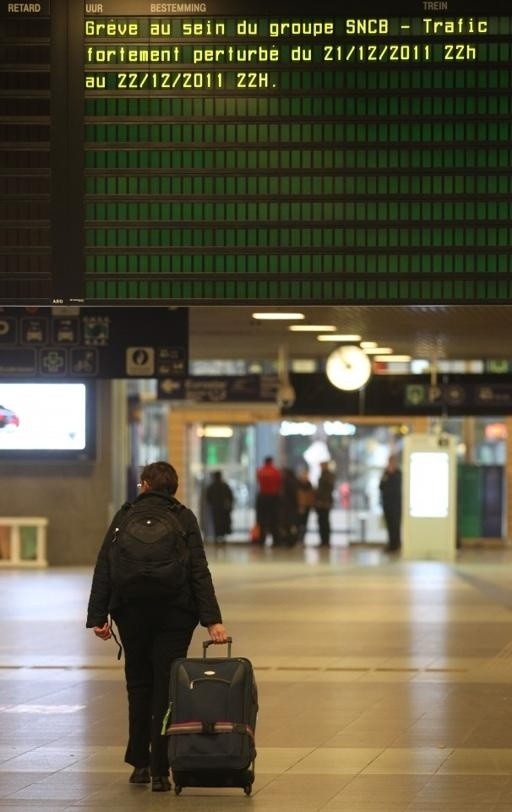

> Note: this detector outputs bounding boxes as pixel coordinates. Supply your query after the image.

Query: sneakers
[151,776,173,792]
[128,766,151,785]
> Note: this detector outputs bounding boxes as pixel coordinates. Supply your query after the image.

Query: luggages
[166,636,260,796]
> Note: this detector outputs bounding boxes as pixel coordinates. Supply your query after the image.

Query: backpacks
[106,499,193,603]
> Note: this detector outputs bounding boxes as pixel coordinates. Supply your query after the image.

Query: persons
[379,455,400,551]
[82,461,226,792]
[206,470,233,544]
[242,453,336,550]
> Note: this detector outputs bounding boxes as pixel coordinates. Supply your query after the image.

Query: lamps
[323,344,373,391]
